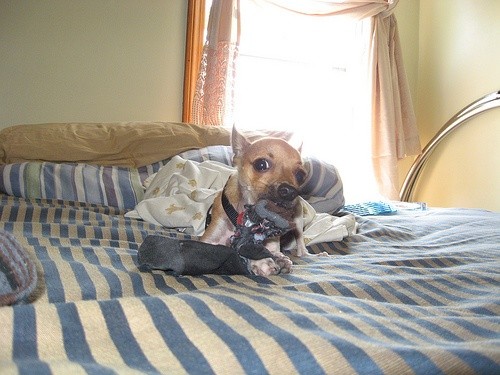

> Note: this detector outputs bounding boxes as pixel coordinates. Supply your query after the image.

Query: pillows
[0,120,222,168]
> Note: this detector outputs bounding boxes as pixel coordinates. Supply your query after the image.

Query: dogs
[197,122,329,277]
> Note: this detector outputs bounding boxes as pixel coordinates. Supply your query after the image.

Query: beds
[1,164,500,375]
